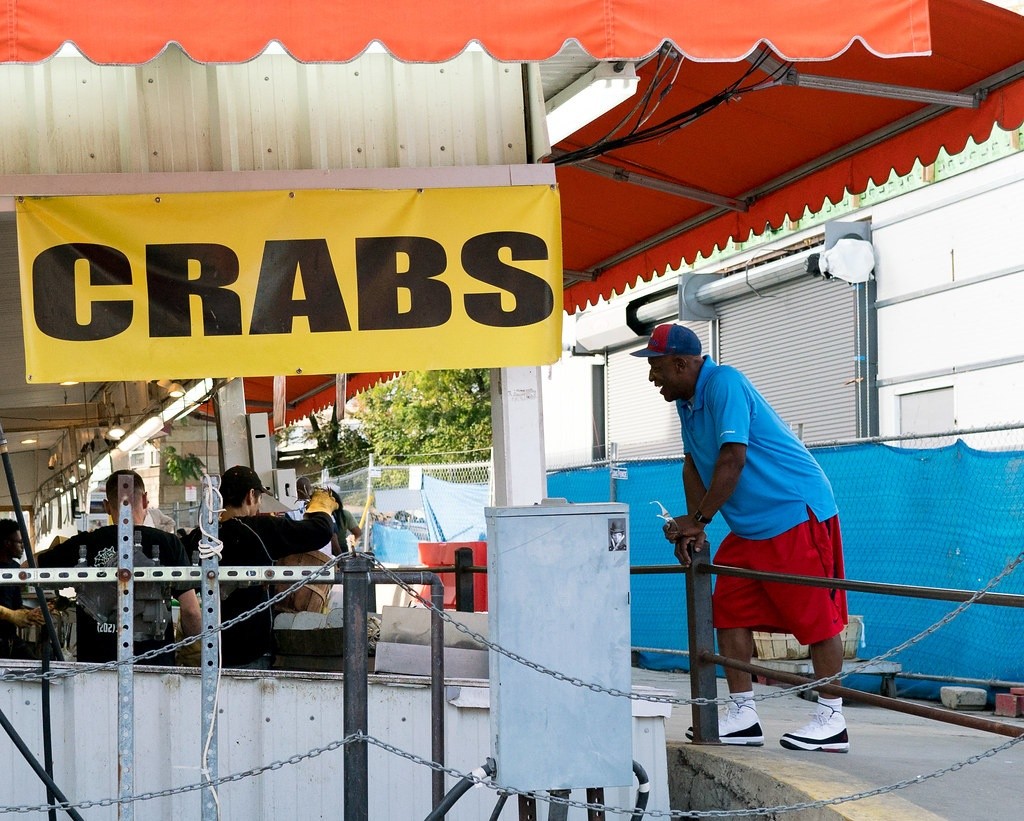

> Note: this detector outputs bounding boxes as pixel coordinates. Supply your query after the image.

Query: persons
[178,465,340,667]
[629,324,849,753]
[143,508,176,533]
[0,518,53,660]
[323,488,362,555]
[21,469,202,667]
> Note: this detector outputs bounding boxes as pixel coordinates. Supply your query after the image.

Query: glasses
[649,501,681,534]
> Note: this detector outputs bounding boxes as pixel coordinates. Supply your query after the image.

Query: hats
[220,465,274,497]
[630,323,701,357]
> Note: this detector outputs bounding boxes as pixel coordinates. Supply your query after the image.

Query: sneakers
[779,700,850,753]
[685,695,765,747]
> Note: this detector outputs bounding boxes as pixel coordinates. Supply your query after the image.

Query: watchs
[694,510,711,524]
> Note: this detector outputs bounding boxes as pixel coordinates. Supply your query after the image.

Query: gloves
[0,605,35,629]
[303,488,339,518]
